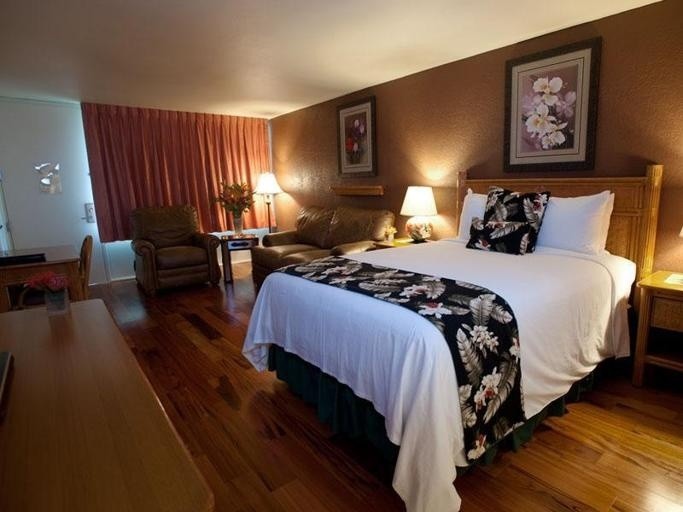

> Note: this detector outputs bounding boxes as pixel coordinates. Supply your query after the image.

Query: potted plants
[384,226,398,241]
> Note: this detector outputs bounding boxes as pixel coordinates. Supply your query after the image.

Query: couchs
[251,204,396,286]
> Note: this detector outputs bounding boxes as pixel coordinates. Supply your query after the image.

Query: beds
[241,163,662,511]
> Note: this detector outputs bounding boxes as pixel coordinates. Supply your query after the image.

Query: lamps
[400,186,438,241]
[252,173,284,233]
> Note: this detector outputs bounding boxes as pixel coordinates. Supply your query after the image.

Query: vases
[43,290,71,316]
[233,211,243,233]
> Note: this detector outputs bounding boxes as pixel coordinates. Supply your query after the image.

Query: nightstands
[632,270,683,388]
[373,237,436,249]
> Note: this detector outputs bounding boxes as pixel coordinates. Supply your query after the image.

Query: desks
[0,299,215,512]
[0,244,81,312]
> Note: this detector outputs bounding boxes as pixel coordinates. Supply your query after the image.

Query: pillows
[453,188,615,256]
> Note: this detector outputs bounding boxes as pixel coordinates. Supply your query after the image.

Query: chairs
[130,205,220,296]
[79,235,93,300]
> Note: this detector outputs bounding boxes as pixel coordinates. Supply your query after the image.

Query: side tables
[220,234,259,283]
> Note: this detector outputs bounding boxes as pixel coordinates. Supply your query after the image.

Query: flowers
[214,181,257,218]
[522,74,576,150]
[25,270,70,308]
[346,120,367,164]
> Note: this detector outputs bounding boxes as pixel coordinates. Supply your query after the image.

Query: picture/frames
[502,36,599,173]
[336,95,377,178]
